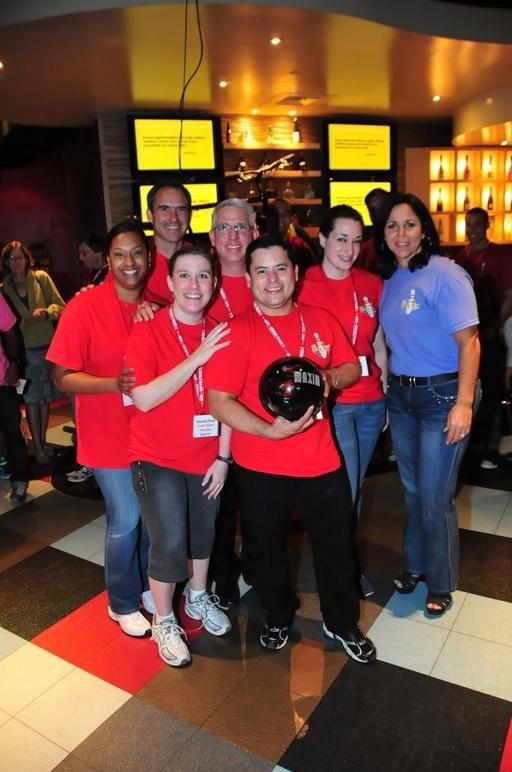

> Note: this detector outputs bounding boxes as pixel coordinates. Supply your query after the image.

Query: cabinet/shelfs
[431,150,511,245]
[222,141,322,233]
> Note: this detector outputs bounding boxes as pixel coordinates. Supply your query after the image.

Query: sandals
[393,572,422,594]
[425,591,450,615]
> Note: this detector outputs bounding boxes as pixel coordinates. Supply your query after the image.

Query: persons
[1,181,511,668]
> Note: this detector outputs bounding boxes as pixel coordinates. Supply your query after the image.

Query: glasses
[211,222,252,232]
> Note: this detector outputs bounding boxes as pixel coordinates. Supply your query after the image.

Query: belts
[388,372,458,386]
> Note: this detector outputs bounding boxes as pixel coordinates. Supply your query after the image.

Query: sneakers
[360,574,374,598]
[322,619,377,664]
[108,590,156,637]
[0,458,26,504]
[260,616,291,650]
[67,465,91,482]
[184,579,237,637]
[151,614,190,667]
[481,460,497,470]
[37,445,53,463]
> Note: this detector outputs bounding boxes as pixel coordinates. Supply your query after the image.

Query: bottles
[437,187,444,212]
[225,121,232,143]
[292,119,301,143]
[437,217,445,245]
[464,186,470,211]
[436,152,444,181]
[487,186,493,211]
[463,155,471,180]
[507,155,512,182]
[487,155,494,178]
